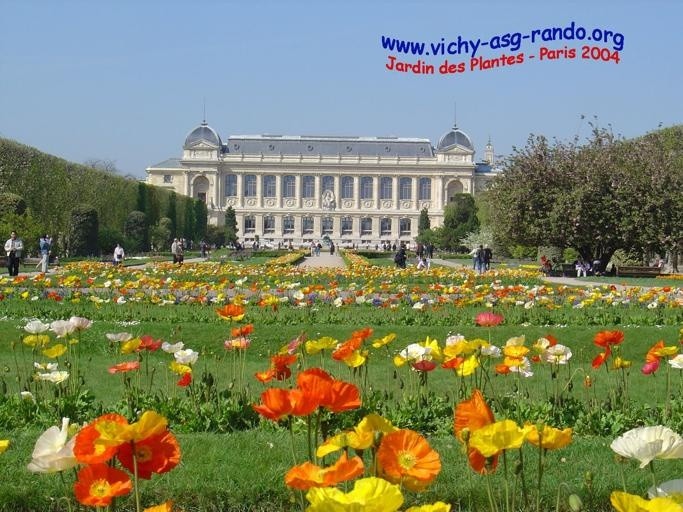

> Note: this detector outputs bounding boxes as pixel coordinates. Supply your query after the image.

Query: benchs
[538,268,592,276]
[617,266,663,278]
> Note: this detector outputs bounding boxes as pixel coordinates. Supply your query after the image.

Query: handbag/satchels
[117,255,122,258]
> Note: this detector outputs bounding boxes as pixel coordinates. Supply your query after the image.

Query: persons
[170,238,180,263]
[467,246,478,270]
[199,239,434,269]
[113,242,124,265]
[177,237,184,261]
[484,244,491,270]
[475,244,485,273]
[3,231,23,276]
[573,255,586,278]
[39,234,50,272]
